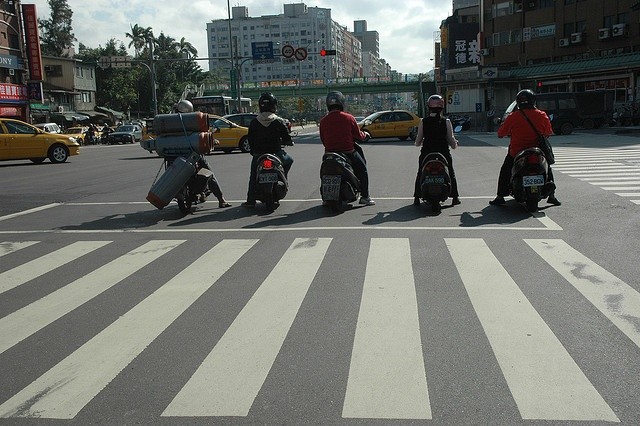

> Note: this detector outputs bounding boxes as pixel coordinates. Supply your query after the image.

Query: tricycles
[572,89,614,129]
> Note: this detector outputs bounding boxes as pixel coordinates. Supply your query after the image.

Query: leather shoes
[219,202,232,207]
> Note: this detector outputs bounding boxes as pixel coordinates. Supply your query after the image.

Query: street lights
[139,62,157,118]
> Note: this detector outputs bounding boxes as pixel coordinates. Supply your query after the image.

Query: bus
[191,96,252,117]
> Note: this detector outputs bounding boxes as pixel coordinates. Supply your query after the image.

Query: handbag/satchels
[519,107,554,164]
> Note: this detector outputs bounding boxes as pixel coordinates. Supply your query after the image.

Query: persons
[319,90,375,206]
[240,92,293,208]
[413,93,461,208]
[87,123,109,144]
[176,99,232,208]
[489,88,562,206]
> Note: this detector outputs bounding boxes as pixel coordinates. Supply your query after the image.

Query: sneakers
[489,198,505,204]
[359,196,376,204]
[242,202,255,206]
[413,199,421,205]
[453,199,462,205]
[547,197,561,205]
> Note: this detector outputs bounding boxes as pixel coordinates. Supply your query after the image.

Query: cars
[223,115,292,134]
[357,110,423,141]
[0,119,79,163]
[65,127,88,146]
[209,115,252,152]
[108,125,143,143]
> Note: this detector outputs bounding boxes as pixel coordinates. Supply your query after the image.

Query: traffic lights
[321,50,337,56]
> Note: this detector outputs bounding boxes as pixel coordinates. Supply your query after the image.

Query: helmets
[259,92,278,113]
[515,88,535,106]
[427,94,446,109]
[178,99,193,112]
[326,90,345,112]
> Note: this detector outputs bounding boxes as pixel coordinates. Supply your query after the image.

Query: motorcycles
[448,115,471,133]
[86,124,112,145]
[611,104,634,126]
[253,139,294,211]
[320,134,370,212]
[509,146,548,211]
[417,140,458,214]
[165,141,211,213]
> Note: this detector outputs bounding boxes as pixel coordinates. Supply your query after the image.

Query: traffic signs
[252,42,273,62]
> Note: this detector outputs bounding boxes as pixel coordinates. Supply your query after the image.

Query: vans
[32,122,61,135]
[502,93,584,135]
